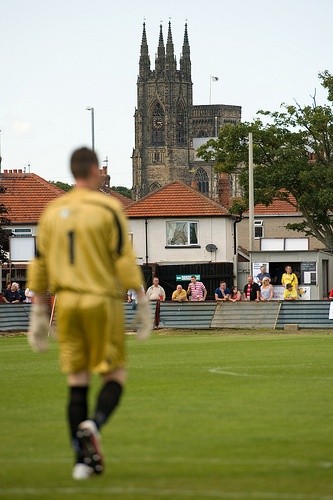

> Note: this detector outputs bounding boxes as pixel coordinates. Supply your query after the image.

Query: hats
[286,284,293,289]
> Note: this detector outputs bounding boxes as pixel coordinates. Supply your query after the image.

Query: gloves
[134,298,152,340]
[28,305,52,352]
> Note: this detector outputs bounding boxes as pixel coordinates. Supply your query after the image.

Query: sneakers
[73,464,92,479]
[77,419,105,475]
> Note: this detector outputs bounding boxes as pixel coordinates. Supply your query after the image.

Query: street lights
[86,105,94,152]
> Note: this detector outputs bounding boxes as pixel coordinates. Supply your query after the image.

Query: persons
[243,277,259,303]
[145,278,165,301]
[172,285,188,302]
[215,281,230,301]
[186,276,207,301]
[256,266,271,285]
[27,146,153,480]
[228,286,241,302]
[127,284,145,303]
[281,266,299,300]
[259,277,273,301]
[0,282,33,303]
